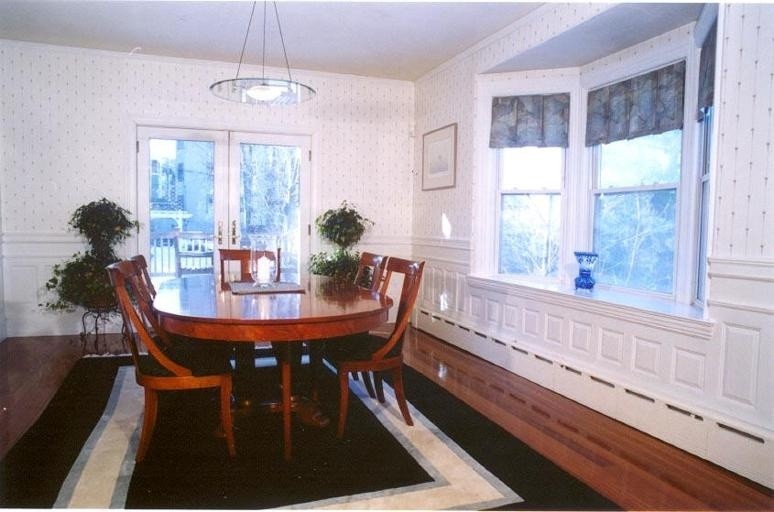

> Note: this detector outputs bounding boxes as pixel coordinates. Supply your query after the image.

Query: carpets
[0,343,623,510]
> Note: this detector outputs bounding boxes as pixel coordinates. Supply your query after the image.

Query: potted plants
[37,195,142,309]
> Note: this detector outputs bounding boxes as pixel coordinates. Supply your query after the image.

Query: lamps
[208,0,316,106]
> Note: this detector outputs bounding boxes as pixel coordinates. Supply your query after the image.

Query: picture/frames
[421,122,458,191]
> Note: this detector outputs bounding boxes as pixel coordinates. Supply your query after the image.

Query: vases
[573,251,599,292]
